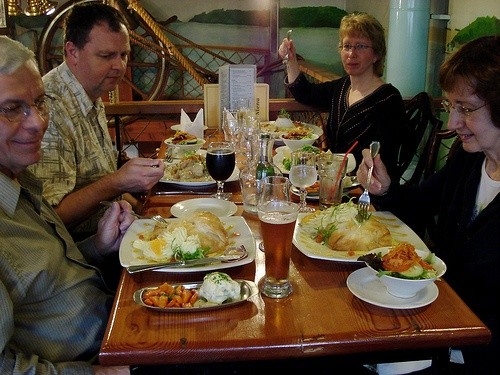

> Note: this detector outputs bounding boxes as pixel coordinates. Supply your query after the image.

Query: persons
[0,35,138,375]
[27,4,165,238]
[355,36,500,375]
[278,13,406,174]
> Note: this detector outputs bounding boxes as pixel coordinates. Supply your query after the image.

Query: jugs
[240,132,283,214]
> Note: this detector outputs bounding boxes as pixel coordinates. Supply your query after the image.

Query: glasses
[337,41,372,51]
[0,94,55,122]
[441,99,489,118]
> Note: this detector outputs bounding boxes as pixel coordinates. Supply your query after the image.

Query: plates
[152,120,362,199]
[133,281,260,311]
[346,267,440,309]
[118,217,257,272]
[169,198,238,218]
[292,211,432,262]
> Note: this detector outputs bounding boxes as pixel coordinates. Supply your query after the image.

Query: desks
[99,132,492,375]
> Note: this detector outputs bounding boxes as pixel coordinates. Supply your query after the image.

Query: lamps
[8,0,59,17]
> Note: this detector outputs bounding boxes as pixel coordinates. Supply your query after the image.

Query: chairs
[394,91,459,187]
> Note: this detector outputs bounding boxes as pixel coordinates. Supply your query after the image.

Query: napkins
[181,107,203,140]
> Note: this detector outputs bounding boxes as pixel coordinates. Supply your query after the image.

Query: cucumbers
[397,261,424,279]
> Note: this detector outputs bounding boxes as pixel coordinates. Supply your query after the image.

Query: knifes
[127,254,242,274]
[155,190,213,196]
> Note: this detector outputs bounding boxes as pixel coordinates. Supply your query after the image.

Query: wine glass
[258,175,291,251]
[223,106,315,214]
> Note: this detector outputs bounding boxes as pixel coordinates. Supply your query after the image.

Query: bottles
[256,134,274,195]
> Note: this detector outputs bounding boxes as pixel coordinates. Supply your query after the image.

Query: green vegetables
[280,107,290,118]
[182,150,207,173]
[171,238,210,260]
[313,205,372,248]
[205,272,231,285]
[282,146,321,170]
[376,252,437,279]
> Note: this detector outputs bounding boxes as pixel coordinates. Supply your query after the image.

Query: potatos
[143,283,199,308]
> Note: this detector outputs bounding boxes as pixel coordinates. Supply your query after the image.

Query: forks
[153,155,174,167]
[98,200,166,224]
[283,29,293,69]
[357,141,380,217]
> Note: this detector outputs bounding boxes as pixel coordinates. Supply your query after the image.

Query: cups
[257,200,300,300]
[316,155,348,209]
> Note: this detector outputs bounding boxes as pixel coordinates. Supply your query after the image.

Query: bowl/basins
[163,137,206,154]
[363,246,447,297]
[280,134,319,150]
